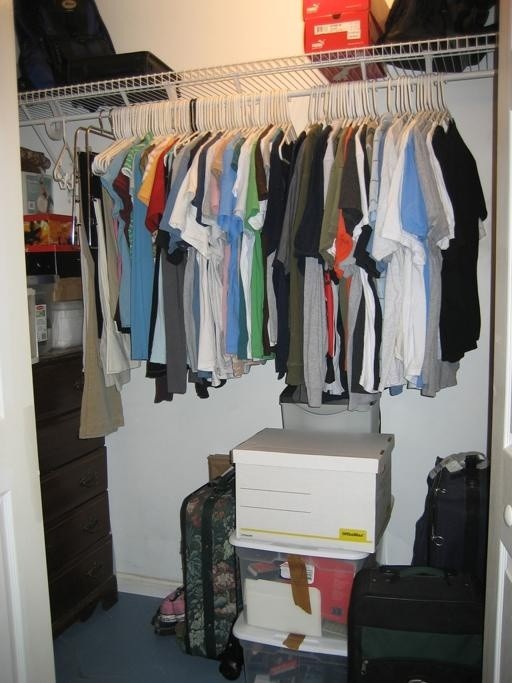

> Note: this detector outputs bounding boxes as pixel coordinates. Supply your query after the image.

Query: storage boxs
[232,607,351,683]
[64,49,180,109]
[230,529,387,645]
[21,171,79,248]
[278,385,381,432]
[25,272,83,361]
[303,0,391,82]
[231,425,395,557]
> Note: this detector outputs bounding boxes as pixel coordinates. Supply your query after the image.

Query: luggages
[348,565,485,682]
[411,454,488,580]
[180,467,239,659]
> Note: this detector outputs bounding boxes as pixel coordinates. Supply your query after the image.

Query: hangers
[49,113,79,189]
[85,73,453,180]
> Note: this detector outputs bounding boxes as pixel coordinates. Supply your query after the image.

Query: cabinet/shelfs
[26,347,118,641]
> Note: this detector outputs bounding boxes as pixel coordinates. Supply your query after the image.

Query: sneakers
[159,587,186,622]
